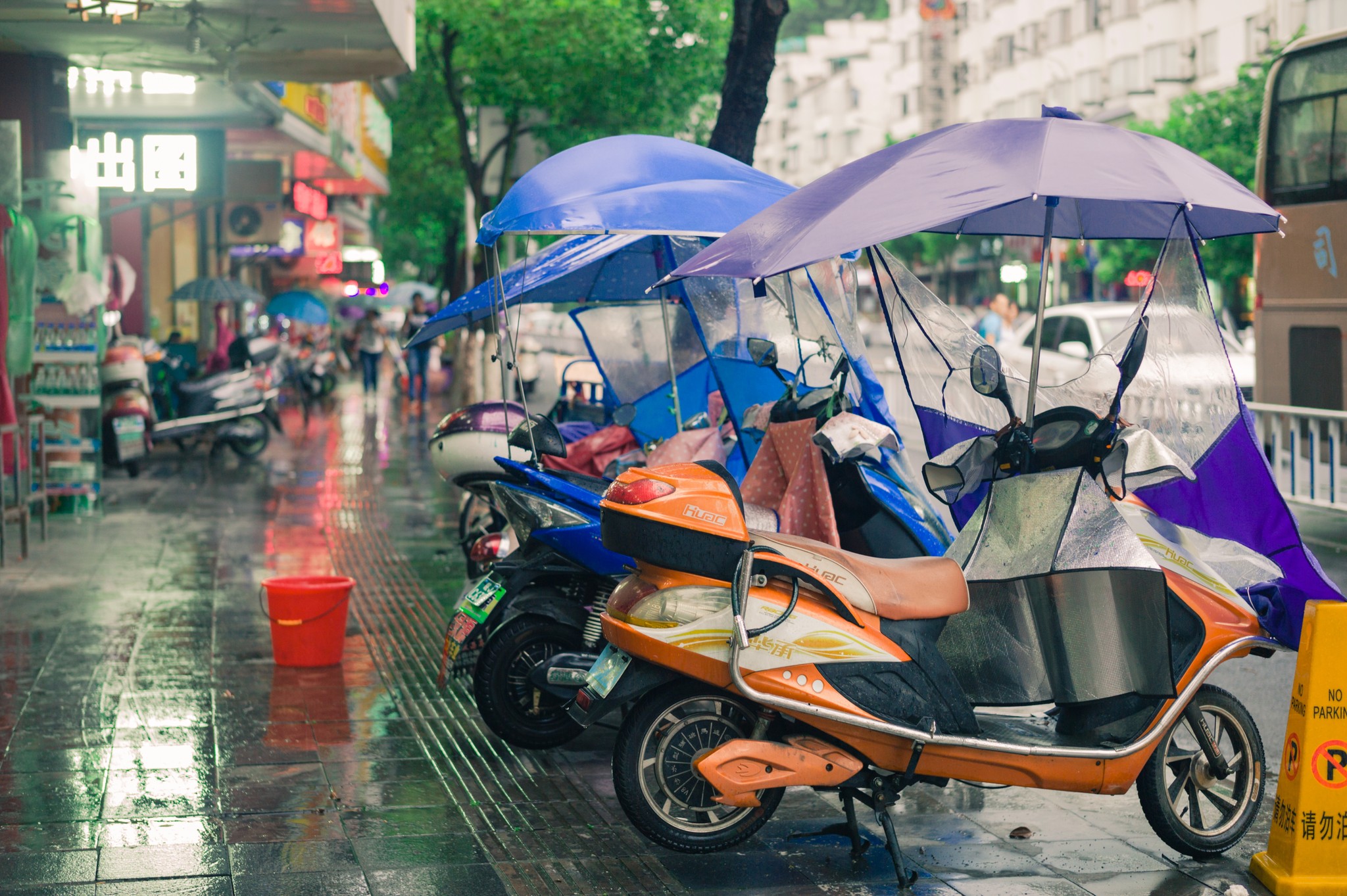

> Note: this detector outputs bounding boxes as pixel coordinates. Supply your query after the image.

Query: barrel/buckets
[259,577,356,667]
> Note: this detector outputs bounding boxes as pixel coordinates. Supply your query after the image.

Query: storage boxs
[599,460,752,579]
[428,400,534,481]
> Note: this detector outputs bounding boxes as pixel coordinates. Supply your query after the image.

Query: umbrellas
[165,274,268,306]
[264,288,336,326]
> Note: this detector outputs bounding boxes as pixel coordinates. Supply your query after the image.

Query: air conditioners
[221,200,284,244]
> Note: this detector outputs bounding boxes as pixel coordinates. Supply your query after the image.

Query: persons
[971,289,1024,353]
[398,293,446,407]
[350,307,386,398]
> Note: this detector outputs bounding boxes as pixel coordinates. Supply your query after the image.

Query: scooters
[97,324,337,480]
[425,311,1265,861]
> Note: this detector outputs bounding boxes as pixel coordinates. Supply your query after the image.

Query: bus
[1252,22,1347,471]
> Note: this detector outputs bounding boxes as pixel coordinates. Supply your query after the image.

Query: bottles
[70,368,79,395]
[90,366,99,396]
[74,323,86,364]
[85,322,98,364]
[79,367,89,396]
[56,369,69,395]
[34,367,45,395]
[54,323,64,363]
[63,324,74,364]
[45,367,55,396]
[33,322,43,362]
[45,322,54,363]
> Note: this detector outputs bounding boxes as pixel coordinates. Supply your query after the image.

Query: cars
[997,300,1256,402]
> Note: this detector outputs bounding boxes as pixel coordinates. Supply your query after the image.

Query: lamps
[65,0,154,26]
